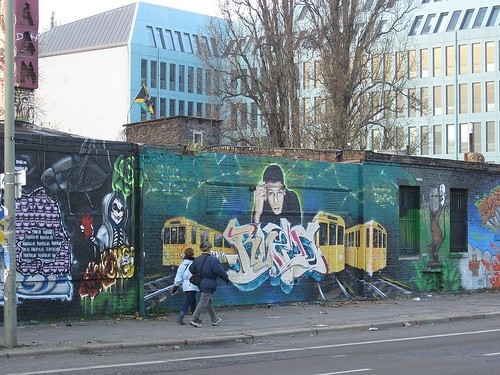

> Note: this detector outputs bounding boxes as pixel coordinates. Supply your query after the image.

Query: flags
[133,85,154,115]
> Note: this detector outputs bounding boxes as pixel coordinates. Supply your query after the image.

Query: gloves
[172,285,178,294]
[226,278,230,284]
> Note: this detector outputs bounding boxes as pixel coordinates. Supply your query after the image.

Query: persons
[252,164,304,225]
[171,243,231,327]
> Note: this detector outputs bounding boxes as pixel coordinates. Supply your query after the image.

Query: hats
[200,241,212,250]
[185,247,194,255]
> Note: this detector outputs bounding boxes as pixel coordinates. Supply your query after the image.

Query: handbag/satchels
[189,272,202,286]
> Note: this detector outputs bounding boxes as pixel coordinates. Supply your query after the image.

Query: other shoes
[194,319,205,324]
[212,318,221,326]
[177,318,187,325]
[190,320,203,327]
